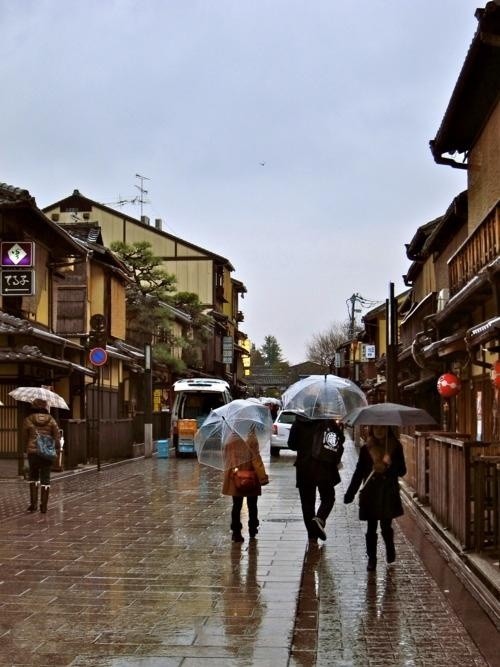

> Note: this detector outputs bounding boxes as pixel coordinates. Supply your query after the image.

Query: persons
[221,411,268,541]
[290,539,326,667]
[19,399,61,514]
[287,394,345,540]
[343,425,406,571]
[222,540,267,665]
[357,567,407,666]
[270,404,278,422]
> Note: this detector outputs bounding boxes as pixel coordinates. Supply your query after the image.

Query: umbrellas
[245,395,283,405]
[195,398,276,471]
[9,386,70,411]
[280,373,368,420]
[343,402,439,458]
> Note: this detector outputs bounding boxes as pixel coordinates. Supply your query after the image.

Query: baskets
[178,419,197,453]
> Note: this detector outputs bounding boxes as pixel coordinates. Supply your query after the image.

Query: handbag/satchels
[233,468,258,489]
[37,434,57,462]
[312,424,341,464]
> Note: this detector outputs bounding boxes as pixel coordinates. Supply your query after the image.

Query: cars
[270,409,306,456]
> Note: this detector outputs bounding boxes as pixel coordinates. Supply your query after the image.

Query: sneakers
[232,529,243,543]
[312,517,326,541]
[249,527,258,537]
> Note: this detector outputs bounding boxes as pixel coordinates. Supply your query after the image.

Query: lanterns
[489,360,500,389]
[436,372,462,399]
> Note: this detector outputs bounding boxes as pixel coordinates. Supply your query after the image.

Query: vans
[170,378,233,458]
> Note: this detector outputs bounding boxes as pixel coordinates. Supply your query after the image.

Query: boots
[366,533,377,571]
[40,485,50,513]
[28,482,38,512]
[381,528,395,563]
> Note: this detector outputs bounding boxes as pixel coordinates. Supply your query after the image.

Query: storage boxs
[178,417,197,436]
[178,436,195,452]
